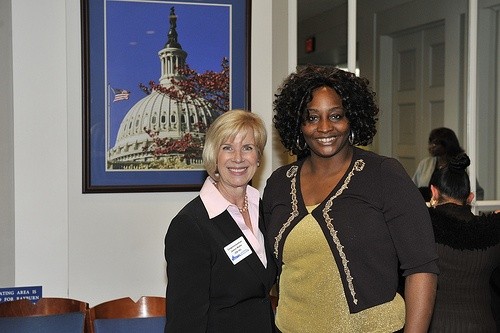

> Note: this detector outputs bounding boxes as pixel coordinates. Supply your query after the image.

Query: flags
[113,88,131,104]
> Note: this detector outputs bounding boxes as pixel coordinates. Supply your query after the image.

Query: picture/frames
[80,0,252,194]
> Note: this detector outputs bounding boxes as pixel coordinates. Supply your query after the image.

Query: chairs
[429,243,500,333]
[86,295,166,333]
[0,298,87,333]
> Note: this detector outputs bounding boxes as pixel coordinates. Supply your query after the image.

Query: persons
[398,127,500,333]
[164,110,277,333]
[262,66,439,333]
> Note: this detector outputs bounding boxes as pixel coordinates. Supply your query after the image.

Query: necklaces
[215,181,248,213]
